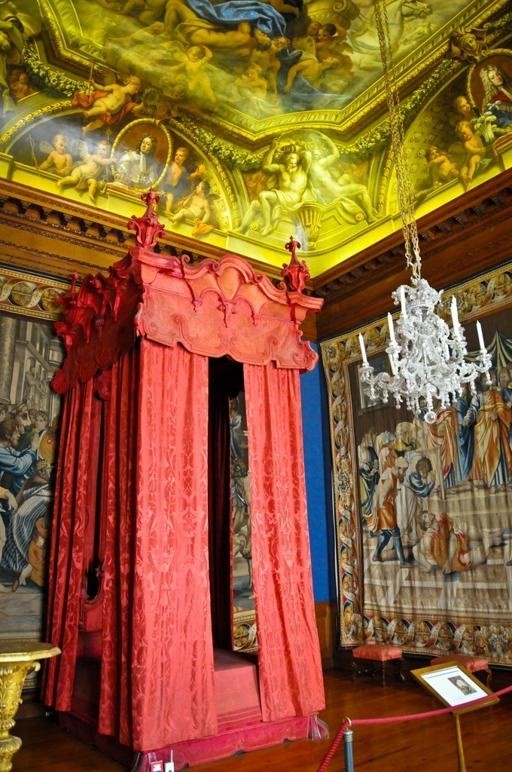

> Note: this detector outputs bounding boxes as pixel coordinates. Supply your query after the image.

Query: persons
[0,411,56,585]
[358,366,512,576]
[229,396,241,475]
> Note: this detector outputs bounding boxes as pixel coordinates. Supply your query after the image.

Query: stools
[347,642,407,689]
[428,651,493,691]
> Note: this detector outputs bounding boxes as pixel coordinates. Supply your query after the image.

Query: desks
[0,636,64,771]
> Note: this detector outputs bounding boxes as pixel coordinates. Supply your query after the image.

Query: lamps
[350,1,495,423]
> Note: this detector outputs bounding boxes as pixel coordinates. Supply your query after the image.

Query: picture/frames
[407,659,500,716]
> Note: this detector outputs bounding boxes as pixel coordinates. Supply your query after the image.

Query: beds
[41,188,335,770]
